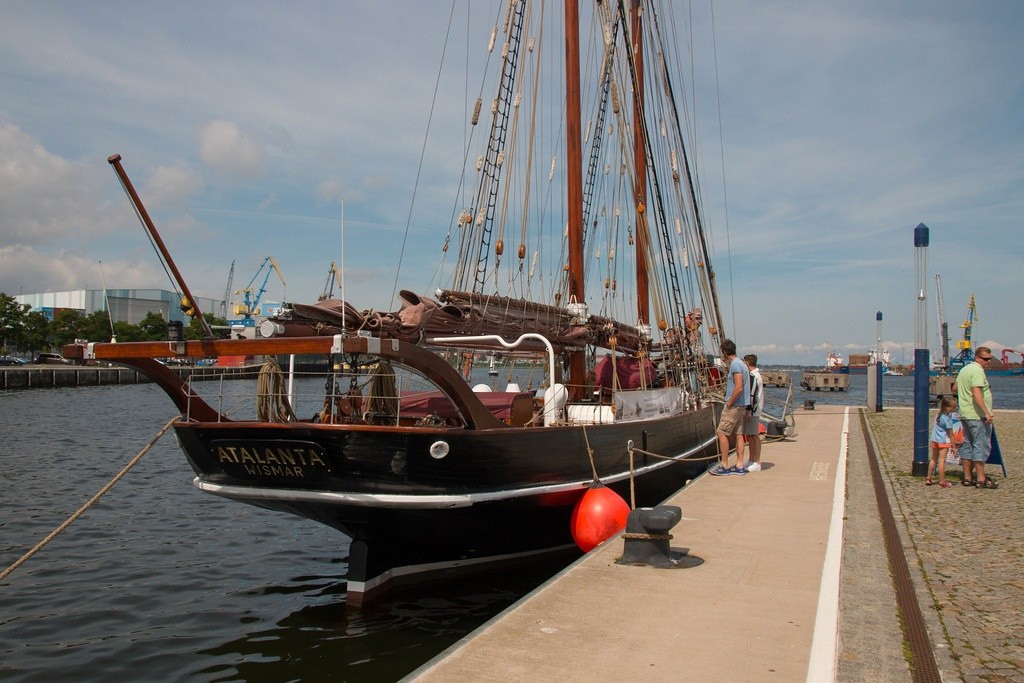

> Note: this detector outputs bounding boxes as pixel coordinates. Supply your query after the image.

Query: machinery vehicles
[215,258,235,338]
[1000,349,1024,368]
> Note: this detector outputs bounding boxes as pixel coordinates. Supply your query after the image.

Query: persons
[709,340,750,476]
[646,371,675,389]
[952,347,999,489]
[925,397,957,488]
[742,354,764,472]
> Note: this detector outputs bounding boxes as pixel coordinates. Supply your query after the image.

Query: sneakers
[708,464,730,476]
[744,461,761,472]
[729,465,745,475]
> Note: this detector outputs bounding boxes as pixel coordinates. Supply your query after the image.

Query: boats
[883,370,903,375]
[62,0,737,604]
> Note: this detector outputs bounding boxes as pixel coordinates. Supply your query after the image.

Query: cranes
[934,273,979,372]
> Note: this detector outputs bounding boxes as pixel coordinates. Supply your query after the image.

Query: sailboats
[488,356,499,377]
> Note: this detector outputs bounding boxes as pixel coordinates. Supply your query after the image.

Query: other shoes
[926,478,933,485]
[937,480,952,488]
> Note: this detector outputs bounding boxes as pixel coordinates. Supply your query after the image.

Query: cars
[0,355,33,365]
[153,356,218,365]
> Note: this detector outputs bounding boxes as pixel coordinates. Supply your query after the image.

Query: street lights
[875,311,885,412]
[912,221,931,479]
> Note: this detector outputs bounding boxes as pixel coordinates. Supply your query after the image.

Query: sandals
[975,476,998,488]
[962,477,976,486]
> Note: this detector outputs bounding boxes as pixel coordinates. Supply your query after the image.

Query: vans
[39,353,70,364]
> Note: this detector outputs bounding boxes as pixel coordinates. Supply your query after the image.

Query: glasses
[978,356,992,361]
